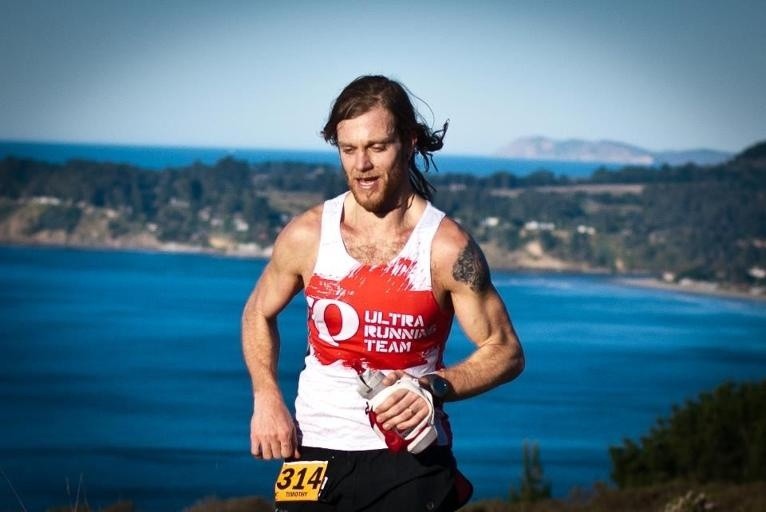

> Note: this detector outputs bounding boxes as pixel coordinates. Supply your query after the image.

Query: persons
[241,75,526,512]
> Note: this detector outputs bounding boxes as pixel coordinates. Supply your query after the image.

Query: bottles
[357,370,439,457]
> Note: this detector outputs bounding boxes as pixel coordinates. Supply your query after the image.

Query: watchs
[420,374,449,402]
